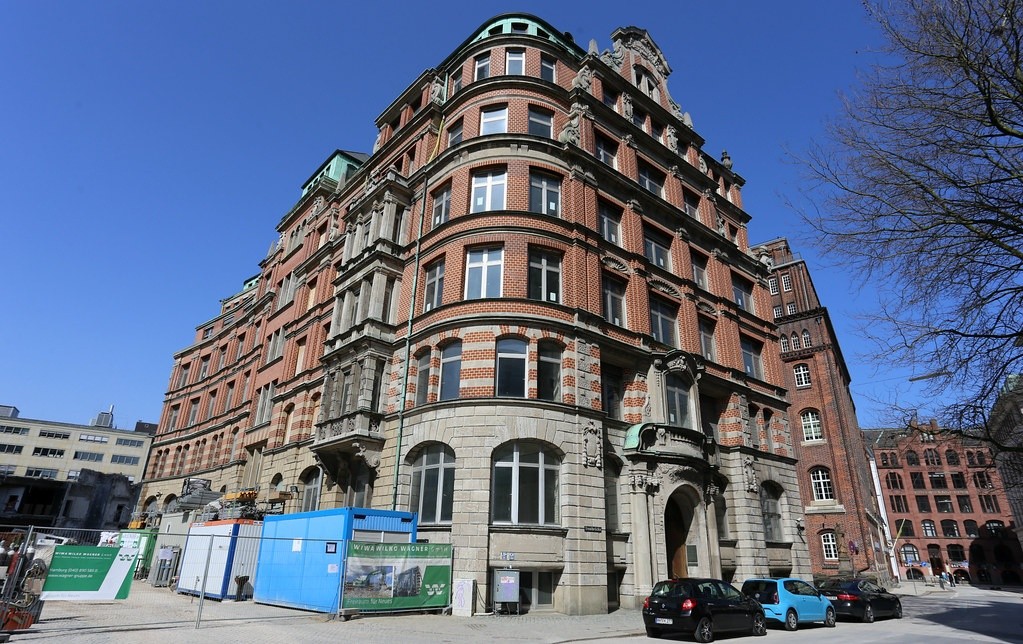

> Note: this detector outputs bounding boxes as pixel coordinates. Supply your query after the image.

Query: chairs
[703,587,711,595]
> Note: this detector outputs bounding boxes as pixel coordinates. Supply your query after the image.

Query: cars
[642,577,767,643]
[740,576,837,632]
[815,576,903,623]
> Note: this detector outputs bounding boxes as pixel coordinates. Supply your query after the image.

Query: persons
[940,570,961,587]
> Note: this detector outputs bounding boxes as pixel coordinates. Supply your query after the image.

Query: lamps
[291,486,298,492]
